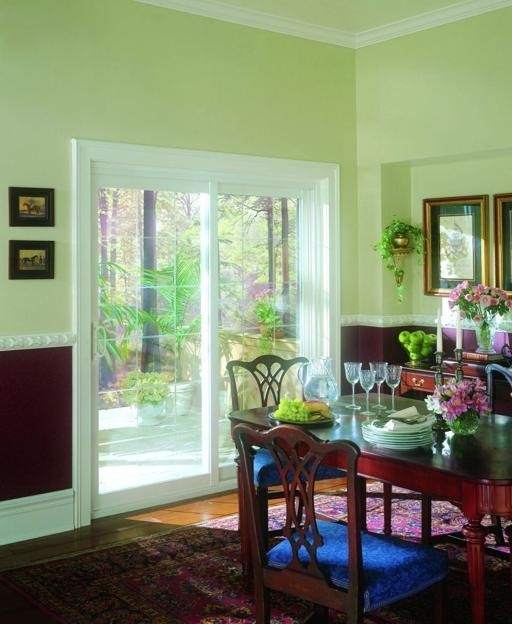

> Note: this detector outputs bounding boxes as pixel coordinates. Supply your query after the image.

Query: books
[461,350,505,363]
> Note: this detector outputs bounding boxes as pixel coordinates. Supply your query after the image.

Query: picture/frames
[6,239,56,281]
[6,185,57,227]
[422,192,512,301]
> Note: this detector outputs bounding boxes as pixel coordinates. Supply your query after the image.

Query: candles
[436,314,466,353]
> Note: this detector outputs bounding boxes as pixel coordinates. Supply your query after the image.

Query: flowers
[424,375,491,419]
[447,279,510,328]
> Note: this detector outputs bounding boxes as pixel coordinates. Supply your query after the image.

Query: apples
[398,330,437,366]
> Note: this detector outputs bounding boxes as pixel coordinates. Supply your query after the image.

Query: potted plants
[371,214,425,286]
[100,229,202,426]
[251,291,290,338]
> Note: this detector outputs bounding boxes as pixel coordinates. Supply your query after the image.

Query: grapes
[272,398,310,422]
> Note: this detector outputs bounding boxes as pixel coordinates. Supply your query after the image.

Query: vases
[445,408,481,436]
[474,316,497,353]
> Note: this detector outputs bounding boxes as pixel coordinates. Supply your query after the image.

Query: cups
[299,358,339,407]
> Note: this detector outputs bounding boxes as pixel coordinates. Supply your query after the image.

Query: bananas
[303,401,330,417]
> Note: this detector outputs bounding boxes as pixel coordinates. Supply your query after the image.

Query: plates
[359,419,435,451]
[267,419,339,430]
[268,410,339,423]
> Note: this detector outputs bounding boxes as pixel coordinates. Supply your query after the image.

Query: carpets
[1,480,512,623]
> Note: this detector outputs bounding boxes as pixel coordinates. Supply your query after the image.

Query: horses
[22,255,38,266]
[23,202,41,216]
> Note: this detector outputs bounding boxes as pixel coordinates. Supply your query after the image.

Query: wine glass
[398,343,434,367]
[343,361,402,417]
